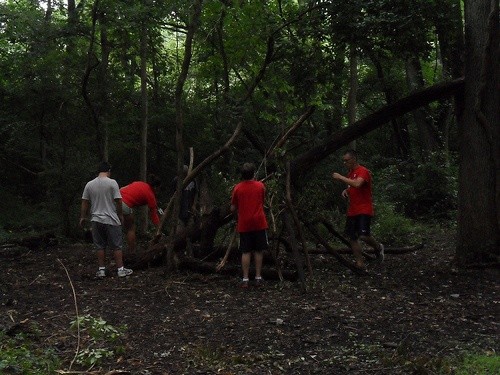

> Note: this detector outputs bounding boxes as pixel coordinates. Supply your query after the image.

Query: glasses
[343,157,353,162]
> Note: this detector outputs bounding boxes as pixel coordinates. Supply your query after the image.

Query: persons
[333,149,385,267]
[119,181,162,254]
[170,164,197,234]
[80,161,133,277]
[230,162,269,288]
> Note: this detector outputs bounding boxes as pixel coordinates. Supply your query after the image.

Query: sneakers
[354,259,364,269]
[96,269,105,277]
[241,281,250,288]
[373,243,384,262]
[118,267,133,277]
[254,279,263,287]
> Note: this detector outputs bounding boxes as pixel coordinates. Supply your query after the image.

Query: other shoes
[127,253,135,261]
